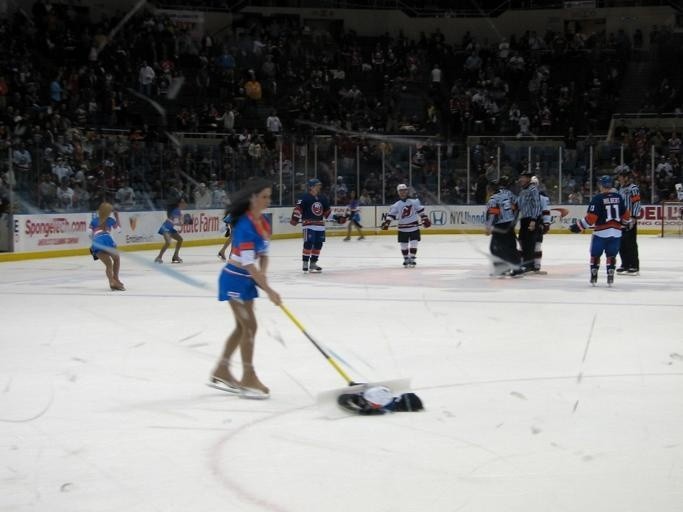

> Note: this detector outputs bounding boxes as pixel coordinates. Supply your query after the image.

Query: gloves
[570,220,584,233]
[382,223,389,230]
[290,216,299,225]
[626,216,635,229]
[423,218,430,227]
[337,214,346,224]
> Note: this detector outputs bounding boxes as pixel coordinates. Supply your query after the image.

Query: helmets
[598,166,632,187]
[492,170,539,189]
[397,184,407,191]
[308,178,321,186]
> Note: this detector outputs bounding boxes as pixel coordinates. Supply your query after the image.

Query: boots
[213,361,241,389]
[241,362,269,393]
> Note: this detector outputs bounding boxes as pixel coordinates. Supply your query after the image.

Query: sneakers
[490,263,540,275]
[303,261,308,270]
[403,257,409,265]
[410,257,416,265]
[311,262,321,269]
[591,268,638,282]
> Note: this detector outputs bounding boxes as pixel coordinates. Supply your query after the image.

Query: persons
[383,182,431,267]
[2,1,682,211]
[340,189,367,242]
[154,196,187,265]
[217,214,231,262]
[208,176,279,399]
[487,163,643,288]
[288,178,344,270]
[90,200,127,292]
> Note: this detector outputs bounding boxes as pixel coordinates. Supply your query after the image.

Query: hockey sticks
[298,211,355,223]
[376,219,425,227]
